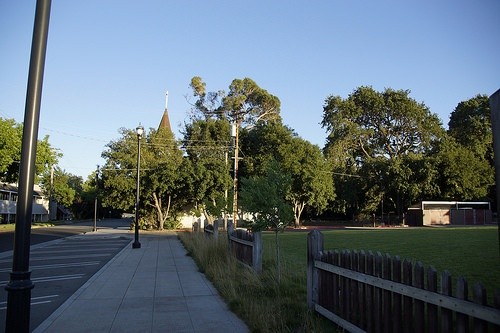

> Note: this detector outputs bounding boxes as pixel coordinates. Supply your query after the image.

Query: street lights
[131,124,144,249]
